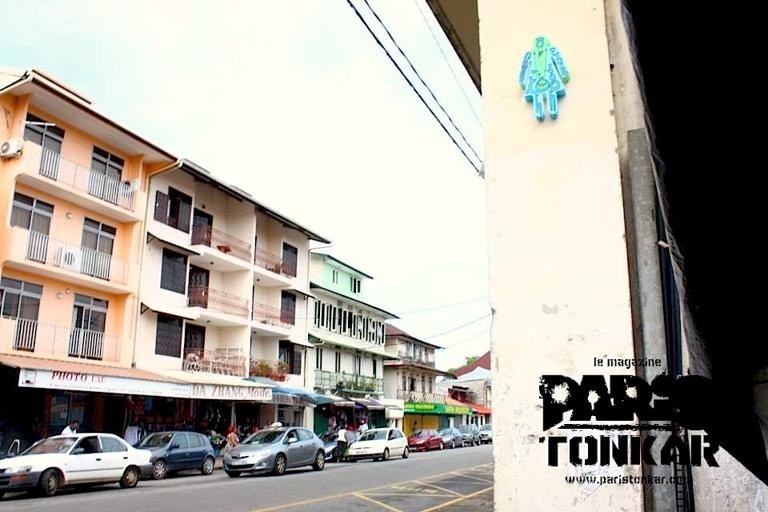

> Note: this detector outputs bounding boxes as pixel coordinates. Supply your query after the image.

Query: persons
[329,418,369,463]
[226,425,241,454]
[209,430,226,457]
[63,420,80,447]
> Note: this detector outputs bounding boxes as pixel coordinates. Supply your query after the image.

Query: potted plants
[251,359,289,381]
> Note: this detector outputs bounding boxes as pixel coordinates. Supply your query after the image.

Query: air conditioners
[1,139,20,159]
[121,178,140,195]
[56,246,82,273]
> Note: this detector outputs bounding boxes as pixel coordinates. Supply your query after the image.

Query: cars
[131,429,217,480]
[222,426,326,479]
[0,432,153,497]
[316,422,493,462]
